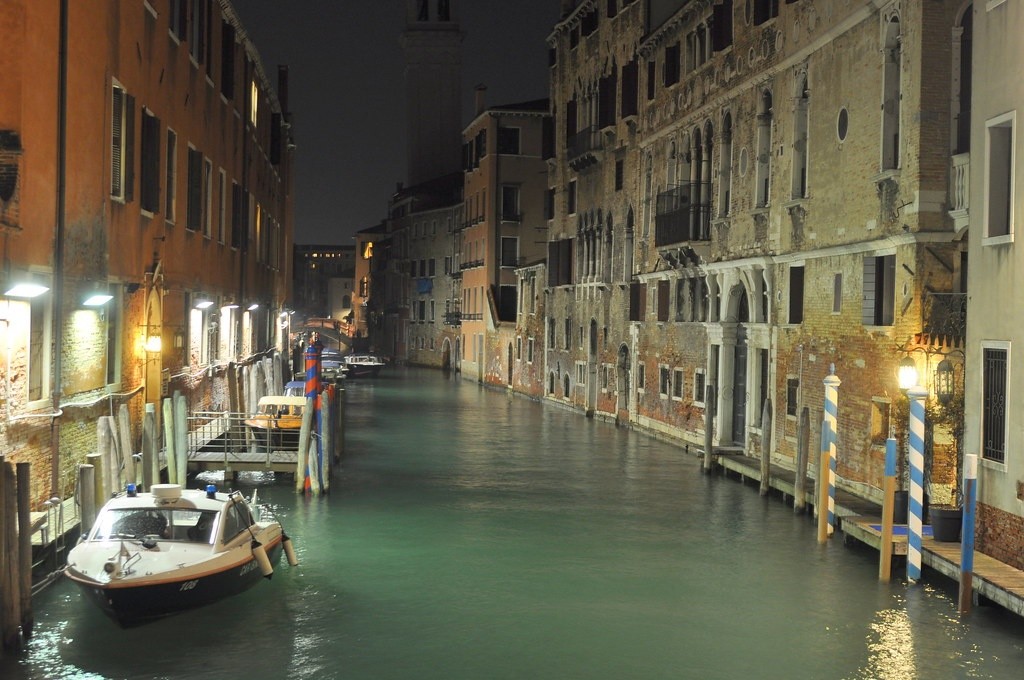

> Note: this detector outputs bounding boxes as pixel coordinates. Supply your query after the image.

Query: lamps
[285,304,295,314]
[245,297,259,311]
[194,298,214,308]
[169,324,187,351]
[224,293,239,309]
[4,278,49,298]
[937,356,955,405]
[82,292,114,307]
[899,351,916,390]
[138,324,161,354]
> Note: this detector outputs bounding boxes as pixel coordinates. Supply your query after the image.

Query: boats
[282,346,386,400]
[63,485,298,629]
[244,395,307,449]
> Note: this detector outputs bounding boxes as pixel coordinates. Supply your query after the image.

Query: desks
[285,381,328,396]
[243,414,301,429]
[258,396,306,414]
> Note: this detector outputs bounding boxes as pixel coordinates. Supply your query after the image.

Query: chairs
[281,428,300,452]
[250,426,279,451]
